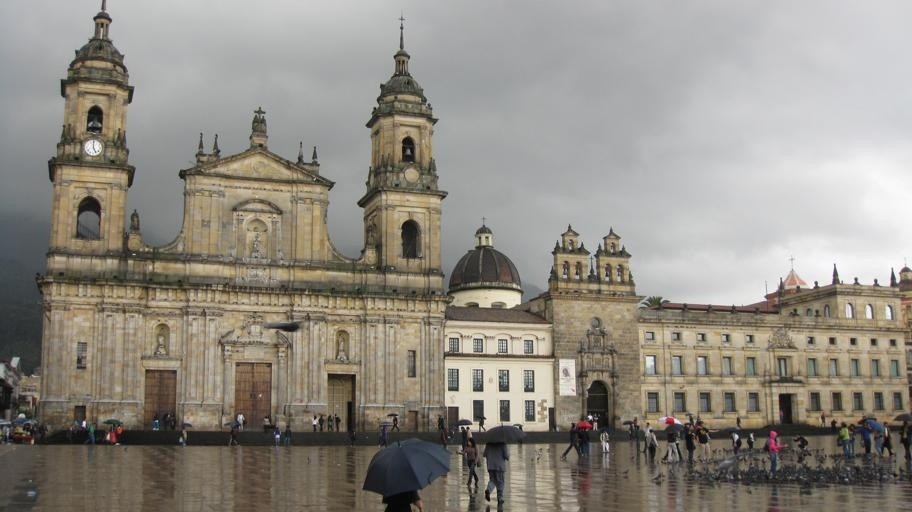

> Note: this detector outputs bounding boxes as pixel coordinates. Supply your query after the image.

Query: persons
[456,438,479,487]
[482,442,510,504]
[382,489,423,512]
[765,483,780,512]
[466,484,479,512]
[558,410,912,483]
[1,411,527,454]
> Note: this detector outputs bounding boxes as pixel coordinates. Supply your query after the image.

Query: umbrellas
[363,437,453,496]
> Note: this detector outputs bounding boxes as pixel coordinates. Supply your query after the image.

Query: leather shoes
[498,500,505,505]
[485,489,490,502]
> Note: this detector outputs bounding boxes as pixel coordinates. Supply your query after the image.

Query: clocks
[84,137,106,157]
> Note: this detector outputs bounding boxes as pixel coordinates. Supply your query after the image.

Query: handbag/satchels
[650,439,656,449]
[477,456,484,468]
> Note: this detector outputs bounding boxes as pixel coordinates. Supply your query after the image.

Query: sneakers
[467,481,471,485]
[475,479,479,485]
